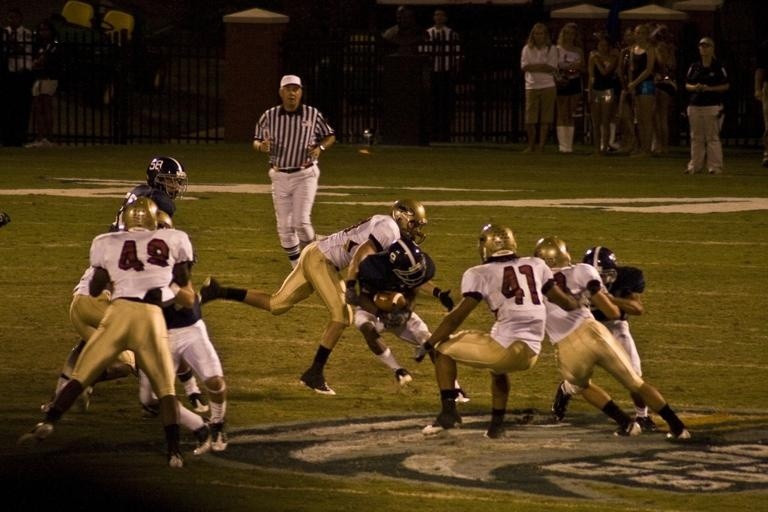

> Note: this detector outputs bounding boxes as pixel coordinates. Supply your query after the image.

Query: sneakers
[551,380,571,420]
[300,368,335,396]
[391,366,413,386]
[521,142,723,175]
[194,274,218,307]
[422,411,462,434]
[162,391,227,470]
[612,414,693,442]
[16,381,97,447]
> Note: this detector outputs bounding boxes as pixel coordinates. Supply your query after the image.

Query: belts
[271,164,316,174]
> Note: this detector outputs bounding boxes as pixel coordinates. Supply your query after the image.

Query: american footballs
[372,290,408,313]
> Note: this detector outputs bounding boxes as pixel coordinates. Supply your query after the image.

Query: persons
[680,38,728,175]
[753,38,768,168]
[519,21,560,156]
[251,71,335,271]
[382,5,416,45]
[420,9,463,141]
[554,23,586,154]
[586,21,675,160]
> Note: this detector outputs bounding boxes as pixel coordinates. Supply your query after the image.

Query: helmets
[583,246,618,283]
[534,233,574,269]
[477,222,518,259]
[121,154,186,232]
[392,198,427,236]
[389,237,428,283]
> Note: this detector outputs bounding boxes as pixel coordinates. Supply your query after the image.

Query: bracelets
[319,144,326,154]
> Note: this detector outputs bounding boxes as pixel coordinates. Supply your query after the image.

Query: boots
[556,125,575,153]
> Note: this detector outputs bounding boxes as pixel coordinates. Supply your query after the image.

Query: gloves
[433,287,455,312]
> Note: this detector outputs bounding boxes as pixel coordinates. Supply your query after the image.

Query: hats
[698,37,714,48]
[280,75,304,89]
